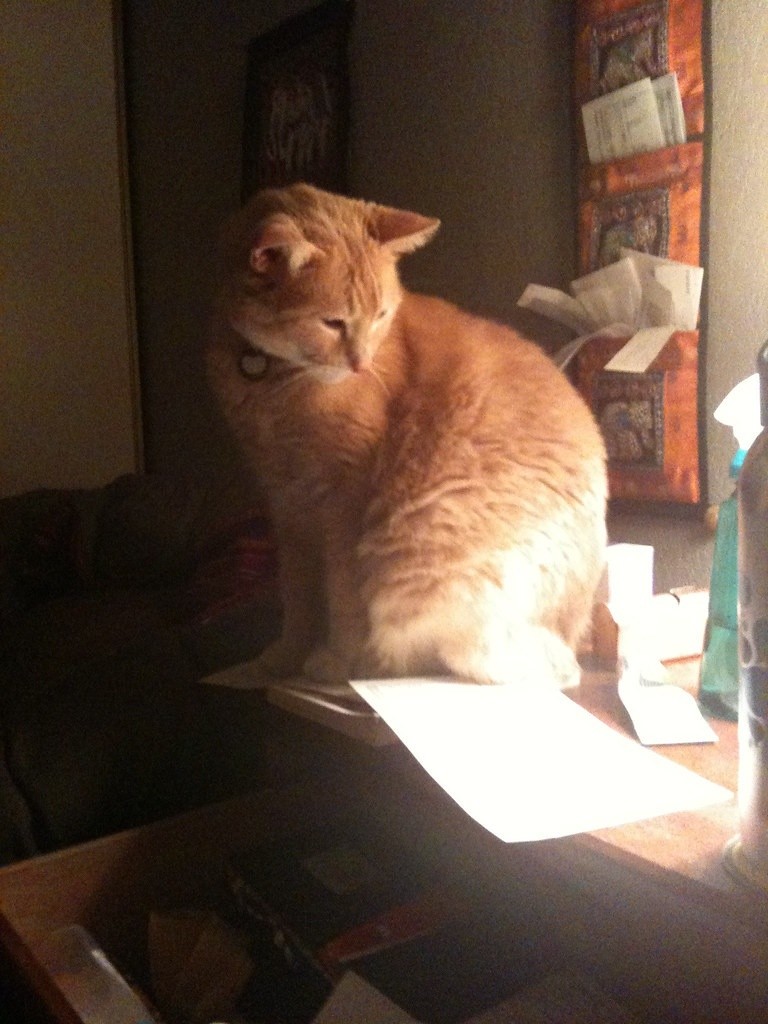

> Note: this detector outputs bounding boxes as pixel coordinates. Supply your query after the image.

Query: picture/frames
[242,1,356,203]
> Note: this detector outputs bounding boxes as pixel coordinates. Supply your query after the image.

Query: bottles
[734,339,767,892]
[698,449,746,721]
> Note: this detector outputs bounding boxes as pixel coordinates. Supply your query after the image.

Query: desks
[272,588,768,929]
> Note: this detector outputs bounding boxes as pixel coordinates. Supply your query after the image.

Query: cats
[200,182,612,691]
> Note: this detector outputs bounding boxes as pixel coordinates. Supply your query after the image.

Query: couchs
[0,475,282,860]
